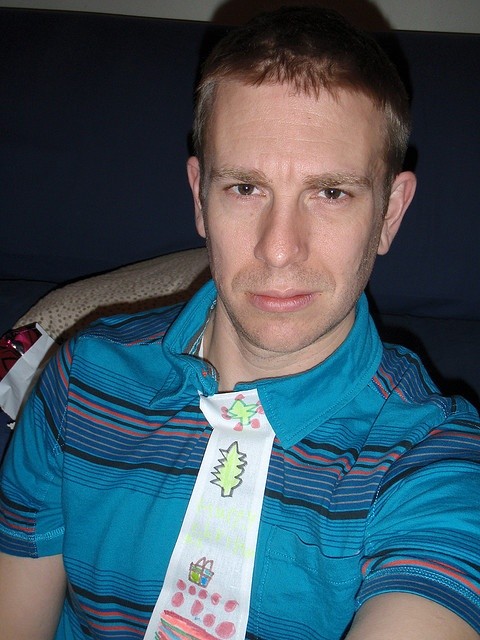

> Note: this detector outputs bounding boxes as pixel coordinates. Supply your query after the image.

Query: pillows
[15,247,211,338]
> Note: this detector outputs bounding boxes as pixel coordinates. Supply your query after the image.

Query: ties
[139,396,277,640]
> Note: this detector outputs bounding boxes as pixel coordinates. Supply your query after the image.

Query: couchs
[3,4,477,404]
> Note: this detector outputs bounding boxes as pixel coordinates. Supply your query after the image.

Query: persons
[0,1,480,640]
